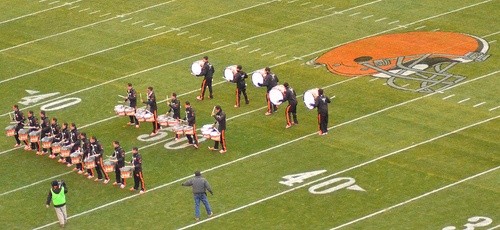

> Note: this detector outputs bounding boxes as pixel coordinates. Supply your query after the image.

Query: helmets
[52,180,57,186]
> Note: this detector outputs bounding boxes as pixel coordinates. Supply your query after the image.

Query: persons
[210,105,227,153]
[141,87,161,137]
[166,93,180,140]
[277,82,299,128]
[182,171,215,222]
[257,67,279,116]
[228,65,249,107]
[194,56,214,100]
[110,141,126,188]
[11,104,111,184]
[46,180,69,227]
[309,89,331,135]
[124,83,139,127]
[182,102,198,149]
[129,147,145,193]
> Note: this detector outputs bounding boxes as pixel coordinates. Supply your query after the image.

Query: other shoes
[286,124,291,128]
[16,144,145,194]
[273,108,277,113]
[292,122,295,125]
[208,212,213,217]
[210,148,218,151]
[157,129,160,132]
[127,122,135,125]
[325,132,327,135]
[208,96,210,99]
[319,133,325,135]
[194,144,198,149]
[187,143,193,145]
[196,217,199,221]
[175,138,180,141]
[234,105,238,107]
[219,150,226,153]
[197,96,203,100]
[152,131,158,136]
[136,125,139,128]
[265,112,272,115]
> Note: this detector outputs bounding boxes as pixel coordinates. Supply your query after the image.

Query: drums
[113,105,135,116]
[157,113,178,126]
[251,68,267,87]
[51,143,62,153]
[201,124,221,141]
[173,123,194,135]
[84,157,96,169]
[224,65,238,81]
[104,161,115,173]
[120,166,133,179]
[18,129,27,140]
[71,153,82,164]
[30,130,41,143]
[269,85,286,106]
[41,137,52,149]
[191,60,205,75]
[136,108,155,122]
[304,88,319,110]
[60,147,70,157]
[5,126,16,136]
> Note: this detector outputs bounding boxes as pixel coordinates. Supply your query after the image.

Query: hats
[195,171,200,175]
